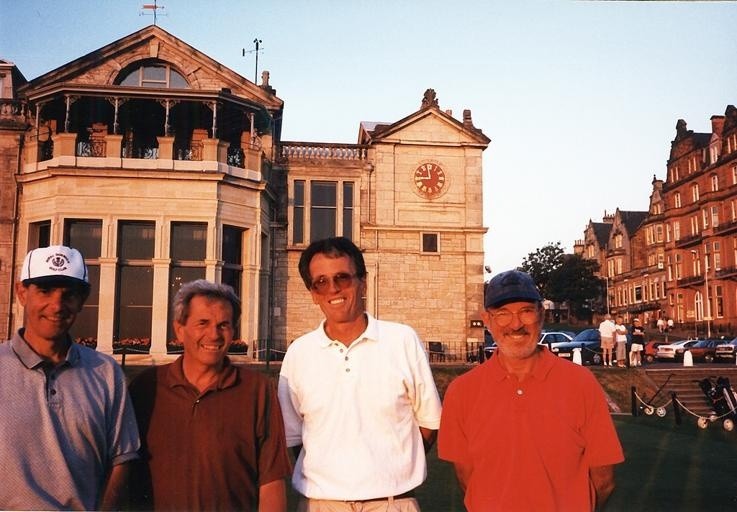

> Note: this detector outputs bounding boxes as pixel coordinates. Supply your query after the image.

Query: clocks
[408,158,452,201]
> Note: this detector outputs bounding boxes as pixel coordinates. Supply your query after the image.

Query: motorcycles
[691,373,736,427]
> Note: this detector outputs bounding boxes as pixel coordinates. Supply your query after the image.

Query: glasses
[311,272,351,294]
[489,309,540,327]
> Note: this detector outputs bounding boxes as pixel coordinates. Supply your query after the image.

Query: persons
[274,235,442,512]
[597,312,615,367]
[629,318,643,366]
[655,317,673,335]
[435,269,626,511]
[125,279,293,512]
[614,314,626,367]
[0,241,143,510]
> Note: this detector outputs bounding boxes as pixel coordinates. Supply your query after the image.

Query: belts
[364,492,416,501]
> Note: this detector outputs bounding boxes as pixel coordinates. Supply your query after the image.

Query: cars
[484,326,737,367]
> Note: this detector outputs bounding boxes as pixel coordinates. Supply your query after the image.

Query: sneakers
[604,362,641,368]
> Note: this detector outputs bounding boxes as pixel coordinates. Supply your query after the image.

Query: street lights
[690,249,711,337]
[590,273,609,314]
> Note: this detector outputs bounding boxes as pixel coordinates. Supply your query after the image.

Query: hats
[485,271,541,307]
[634,318,639,321]
[21,246,89,286]
